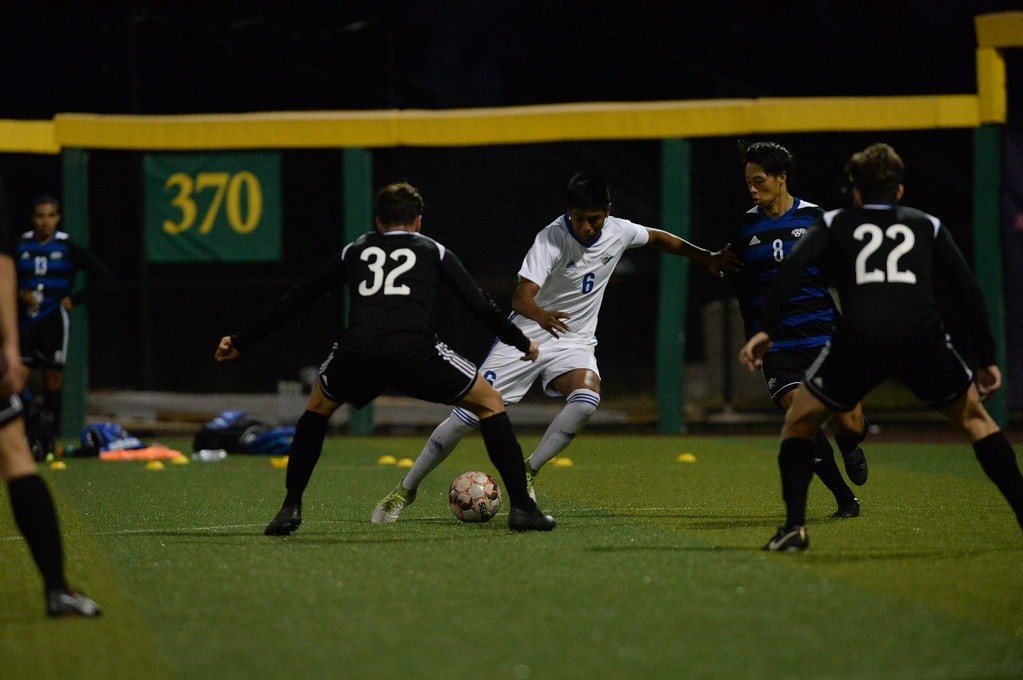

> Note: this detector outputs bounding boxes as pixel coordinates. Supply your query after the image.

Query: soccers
[446,471,503,522]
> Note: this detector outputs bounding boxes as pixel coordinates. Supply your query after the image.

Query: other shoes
[45,592,101,617]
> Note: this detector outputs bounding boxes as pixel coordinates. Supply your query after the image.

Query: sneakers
[265,506,301,537]
[834,432,869,486]
[509,507,556,532]
[371,477,419,524]
[761,526,810,554]
[833,498,860,519]
[523,460,537,503]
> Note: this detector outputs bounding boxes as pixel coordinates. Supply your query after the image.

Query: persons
[215,182,556,534]
[0,254,105,619]
[371,168,735,532]
[12,196,100,438]
[730,142,1023,553]
